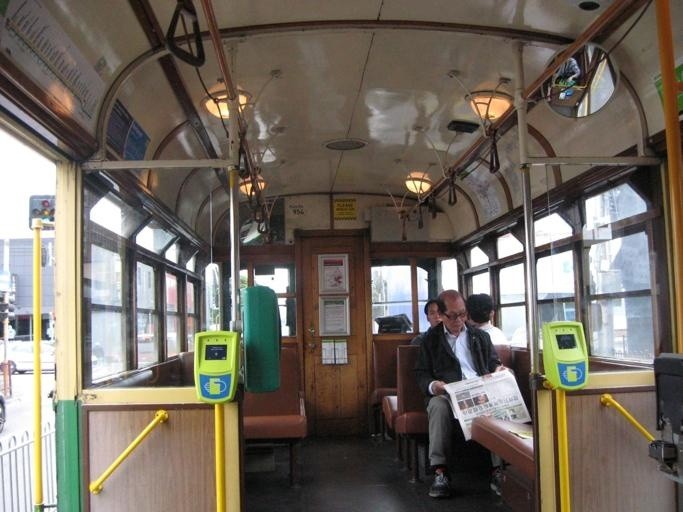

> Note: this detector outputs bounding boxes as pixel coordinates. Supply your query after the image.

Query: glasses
[444,312,466,321]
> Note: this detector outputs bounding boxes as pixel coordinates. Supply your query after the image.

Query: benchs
[176,338,310,490]
[370,327,670,509]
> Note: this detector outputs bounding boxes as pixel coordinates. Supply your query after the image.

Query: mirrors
[239,215,269,249]
[541,40,620,119]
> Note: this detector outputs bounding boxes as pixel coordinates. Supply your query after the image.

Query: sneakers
[491,474,504,494]
[428,473,450,498]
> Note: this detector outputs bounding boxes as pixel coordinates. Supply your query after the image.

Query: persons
[412,290,510,500]
[550,57,580,87]
[404,299,443,346]
[465,293,509,347]
